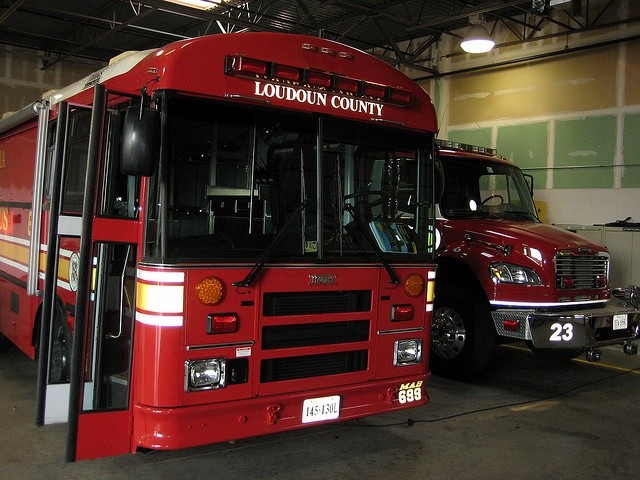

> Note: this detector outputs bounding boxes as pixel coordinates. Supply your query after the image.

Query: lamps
[461,15,495,54]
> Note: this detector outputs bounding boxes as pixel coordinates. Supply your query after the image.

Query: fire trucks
[349,140,640,370]
[1,32,445,462]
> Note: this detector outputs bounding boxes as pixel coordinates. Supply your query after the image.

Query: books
[368,220,418,254]
